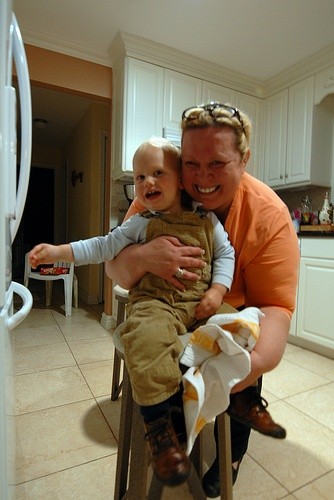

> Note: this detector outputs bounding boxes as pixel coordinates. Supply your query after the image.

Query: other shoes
[201,455,244,498]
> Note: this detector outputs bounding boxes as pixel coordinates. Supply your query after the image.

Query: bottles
[301,194,312,225]
[318,191,332,225]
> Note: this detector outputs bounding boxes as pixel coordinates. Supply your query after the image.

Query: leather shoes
[225,397,287,440]
[142,414,192,487]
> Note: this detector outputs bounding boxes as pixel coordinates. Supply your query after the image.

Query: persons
[104,100,298,497]
[29,136,287,484]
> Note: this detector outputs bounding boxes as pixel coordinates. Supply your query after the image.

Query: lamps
[34,119,48,129]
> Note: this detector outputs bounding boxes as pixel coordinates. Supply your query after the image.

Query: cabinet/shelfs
[295,236,334,350]
[236,92,263,183]
[110,56,164,175]
[164,67,236,144]
[264,63,334,192]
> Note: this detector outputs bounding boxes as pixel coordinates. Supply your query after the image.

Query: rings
[175,270,184,278]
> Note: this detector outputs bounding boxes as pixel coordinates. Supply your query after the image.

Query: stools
[110,284,232,500]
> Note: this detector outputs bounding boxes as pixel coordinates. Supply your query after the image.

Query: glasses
[181,103,247,138]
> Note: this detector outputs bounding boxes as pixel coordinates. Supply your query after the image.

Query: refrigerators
[0,0,34,499]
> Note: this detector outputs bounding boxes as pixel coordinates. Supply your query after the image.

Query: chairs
[23,250,75,317]
